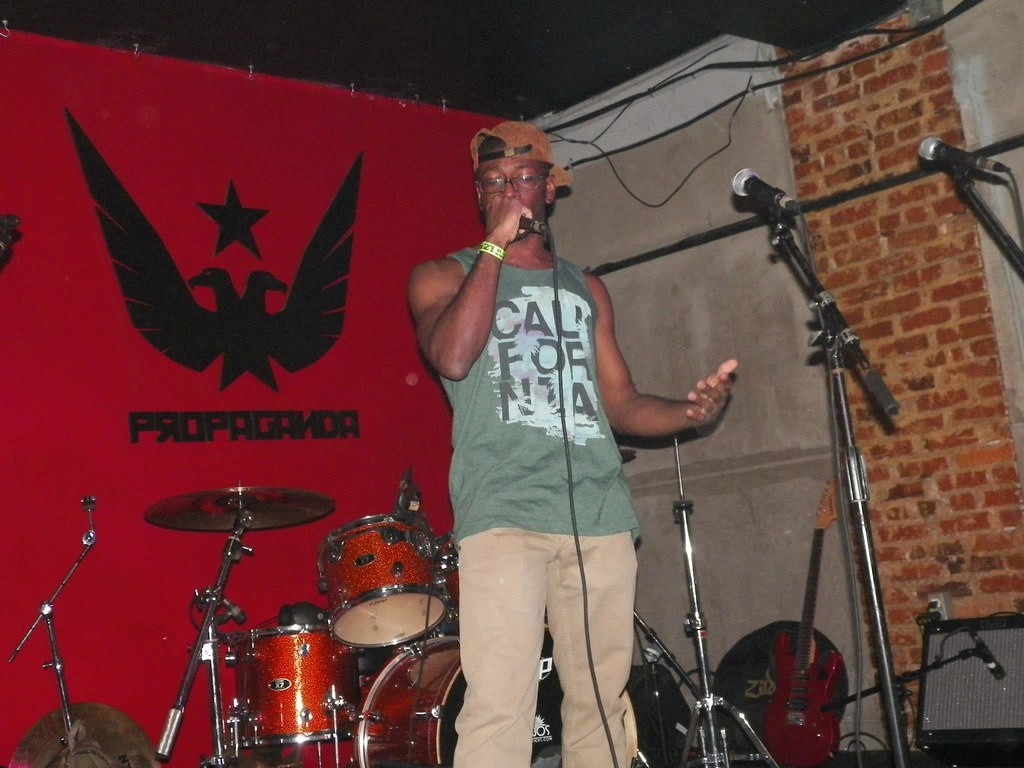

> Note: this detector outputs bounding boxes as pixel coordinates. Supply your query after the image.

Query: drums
[227,622,362,750]
[314,513,450,649]
[352,634,639,768]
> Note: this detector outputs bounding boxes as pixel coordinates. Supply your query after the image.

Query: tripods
[671,433,779,768]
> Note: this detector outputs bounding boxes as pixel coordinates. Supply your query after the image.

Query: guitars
[759,477,844,768]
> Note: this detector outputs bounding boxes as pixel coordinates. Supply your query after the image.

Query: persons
[406,122,738,767]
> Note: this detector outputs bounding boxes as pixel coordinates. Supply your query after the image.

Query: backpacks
[713,619,847,752]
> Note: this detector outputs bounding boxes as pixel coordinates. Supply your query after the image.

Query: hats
[470,121,571,187]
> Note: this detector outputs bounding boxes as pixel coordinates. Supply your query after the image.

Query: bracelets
[477,241,504,261]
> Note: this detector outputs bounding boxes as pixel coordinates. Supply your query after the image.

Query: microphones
[393,470,411,515]
[219,594,247,625]
[968,625,1005,680]
[518,216,547,233]
[732,168,801,212]
[919,136,1010,173]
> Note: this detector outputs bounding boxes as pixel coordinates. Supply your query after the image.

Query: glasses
[476,174,550,195]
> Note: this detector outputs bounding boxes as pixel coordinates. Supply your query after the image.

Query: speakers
[914,613,1024,768]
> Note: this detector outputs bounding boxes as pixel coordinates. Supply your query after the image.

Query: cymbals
[7,701,163,768]
[142,485,336,533]
[620,448,637,462]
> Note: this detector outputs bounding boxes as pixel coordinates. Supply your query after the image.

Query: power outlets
[928,591,953,620]
